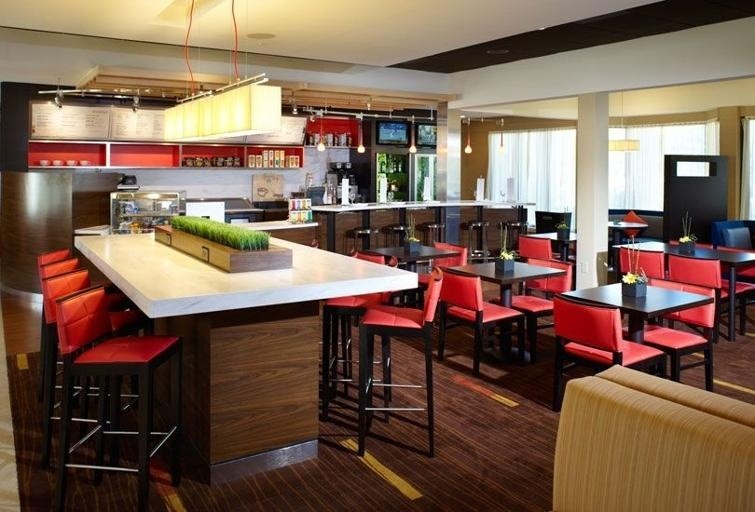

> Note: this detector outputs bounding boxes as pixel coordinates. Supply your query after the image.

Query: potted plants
[154,216,292,273]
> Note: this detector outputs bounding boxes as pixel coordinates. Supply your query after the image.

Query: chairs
[35,249,189,468]
[49,285,185,512]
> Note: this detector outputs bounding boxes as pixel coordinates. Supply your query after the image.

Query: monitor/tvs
[415,121,437,148]
[376,120,410,145]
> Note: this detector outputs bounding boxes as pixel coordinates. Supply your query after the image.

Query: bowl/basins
[40,160,88,166]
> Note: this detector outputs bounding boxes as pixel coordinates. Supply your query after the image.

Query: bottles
[322,183,337,205]
[381,154,402,173]
[305,172,314,188]
[184,156,240,167]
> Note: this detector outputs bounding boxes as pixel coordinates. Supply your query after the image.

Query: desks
[74,234,417,486]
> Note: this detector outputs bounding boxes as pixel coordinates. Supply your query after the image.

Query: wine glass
[500,191,505,202]
[349,192,355,206]
[388,192,394,202]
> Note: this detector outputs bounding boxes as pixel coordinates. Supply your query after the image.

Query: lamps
[357,123,365,152]
[409,123,417,152]
[316,108,327,153]
[464,126,472,153]
[163,1,282,142]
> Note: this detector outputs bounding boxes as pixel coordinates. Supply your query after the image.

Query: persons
[389,180,406,201]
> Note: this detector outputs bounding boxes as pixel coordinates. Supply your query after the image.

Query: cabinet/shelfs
[27,140,304,170]
